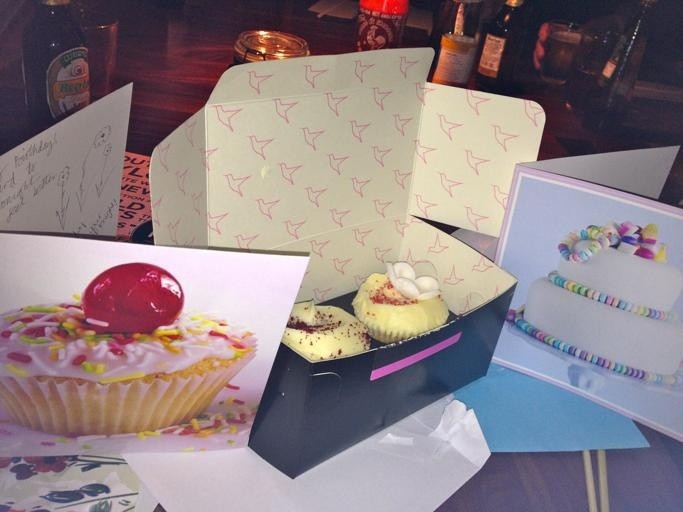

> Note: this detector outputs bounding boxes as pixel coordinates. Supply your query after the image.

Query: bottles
[475,1,530,97]
[579,1,653,134]
[430,0,480,87]
[353,1,410,52]
[22,0,93,130]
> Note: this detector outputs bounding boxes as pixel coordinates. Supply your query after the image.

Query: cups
[86,12,118,98]
[580,26,617,76]
[533,17,580,87]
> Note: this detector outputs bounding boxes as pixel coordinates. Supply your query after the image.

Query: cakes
[284,303,368,361]
[354,272,450,344]
[0,301,258,439]
[507,220,682,391]
